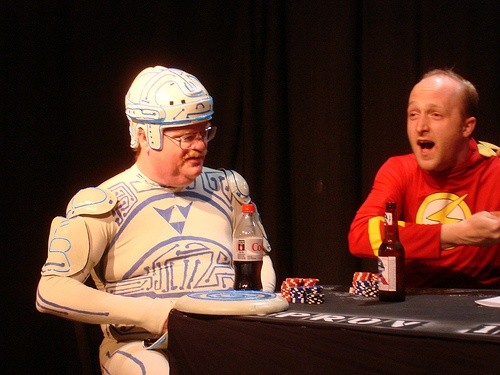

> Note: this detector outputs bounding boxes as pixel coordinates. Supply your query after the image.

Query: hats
[125,65,213,151]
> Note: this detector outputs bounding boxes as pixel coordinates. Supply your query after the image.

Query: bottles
[232,204,263,291]
[378,203,404,301]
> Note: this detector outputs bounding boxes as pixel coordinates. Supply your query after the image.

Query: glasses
[163,127,216,150]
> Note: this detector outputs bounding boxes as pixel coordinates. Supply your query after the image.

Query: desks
[167,284,500,375]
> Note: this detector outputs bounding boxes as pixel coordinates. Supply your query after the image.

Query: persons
[34,66,277,375]
[348,69,500,289]
